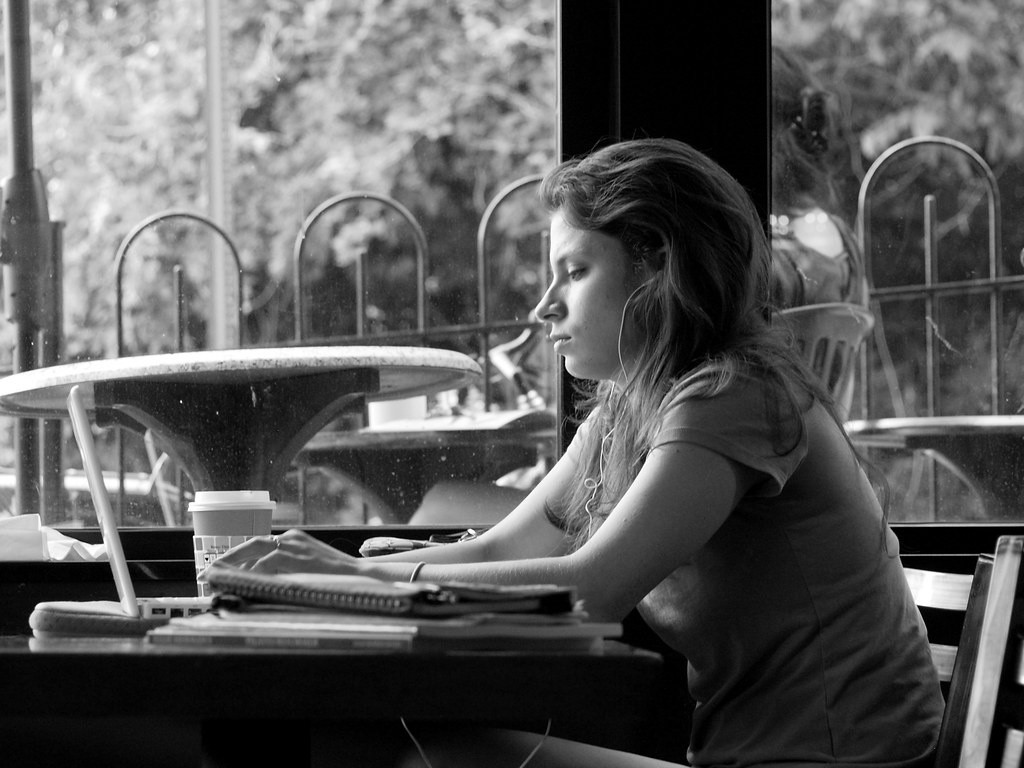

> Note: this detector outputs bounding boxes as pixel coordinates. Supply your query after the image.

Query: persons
[197,140,947,768]
[769,46,868,387]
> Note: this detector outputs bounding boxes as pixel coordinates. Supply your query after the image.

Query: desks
[297,429,545,522]
[0,346,481,612]
[0,607,661,768]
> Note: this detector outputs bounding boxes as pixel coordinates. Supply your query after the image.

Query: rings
[272,535,281,549]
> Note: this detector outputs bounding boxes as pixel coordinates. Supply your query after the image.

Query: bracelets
[410,561,424,582]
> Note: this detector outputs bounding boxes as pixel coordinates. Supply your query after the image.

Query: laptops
[66,386,224,621]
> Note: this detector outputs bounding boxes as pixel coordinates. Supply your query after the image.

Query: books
[151,561,621,656]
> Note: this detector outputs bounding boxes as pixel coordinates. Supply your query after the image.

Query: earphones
[641,271,663,288]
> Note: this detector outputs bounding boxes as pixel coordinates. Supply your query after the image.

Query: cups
[188,489,276,599]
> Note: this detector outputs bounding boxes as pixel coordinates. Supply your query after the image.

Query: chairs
[771,300,878,426]
[937,529,1024,767]
